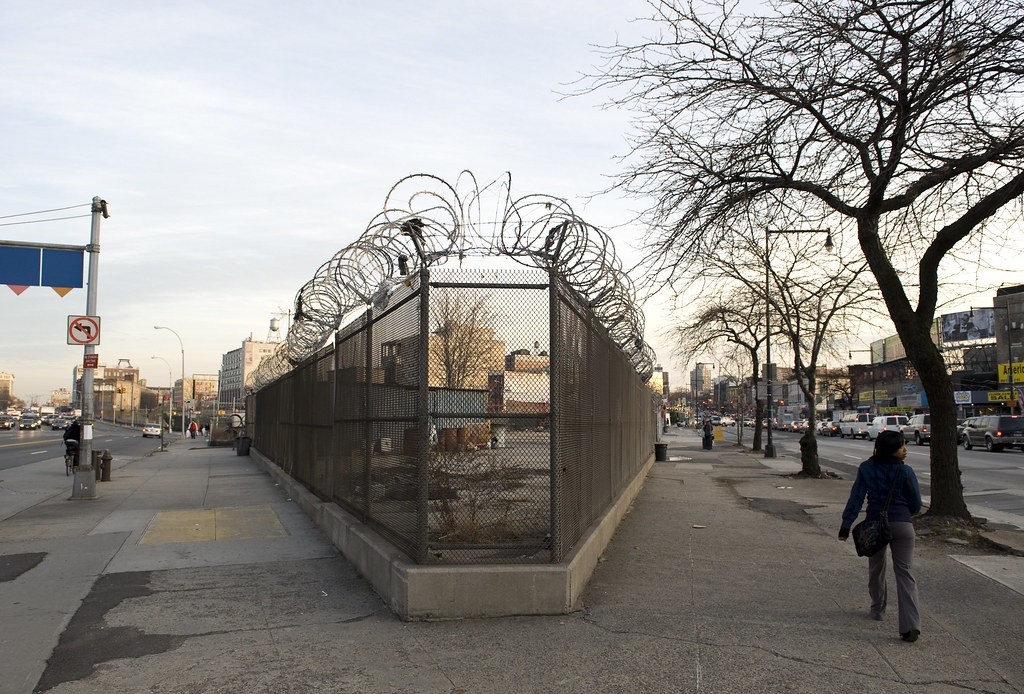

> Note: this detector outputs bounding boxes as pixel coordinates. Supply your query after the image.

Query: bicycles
[65,452,77,476]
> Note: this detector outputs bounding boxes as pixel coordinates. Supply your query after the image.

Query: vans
[839,413,876,440]
[866,416,910,441]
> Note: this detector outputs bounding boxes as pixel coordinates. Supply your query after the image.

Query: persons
[702,419,714,450]
[63,422,81,469]
[837,430,922,643]
[187,420,211,440]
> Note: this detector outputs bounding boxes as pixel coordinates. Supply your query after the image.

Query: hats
[72,421,80,425]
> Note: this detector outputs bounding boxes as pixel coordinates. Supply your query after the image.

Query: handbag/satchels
[851,514,892,557]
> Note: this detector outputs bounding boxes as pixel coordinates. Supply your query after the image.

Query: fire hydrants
[100,448,113,481]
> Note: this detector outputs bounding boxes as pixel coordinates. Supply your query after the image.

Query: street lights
[763,227,835,458]
[969,306,1014,413]
[695,362,716,425]
[848,350,876,413]
[152,327,185,435]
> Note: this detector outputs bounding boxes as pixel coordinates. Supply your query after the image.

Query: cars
[141,423,162,437]
[789,420,827,434]
[0,414,79,430]
[693,413,792,430]
[820,421,841,437]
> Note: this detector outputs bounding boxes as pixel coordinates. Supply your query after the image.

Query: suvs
[957,417,978,444]
[962,414,1024,452]
[899,414,932,445]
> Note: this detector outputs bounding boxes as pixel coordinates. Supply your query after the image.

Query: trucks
[30,407,40,417]
[11,411,21,421]
[39,407,55,423]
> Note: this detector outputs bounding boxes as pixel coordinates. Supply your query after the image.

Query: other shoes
[903,630,918,642]
[870,609,885,620]
